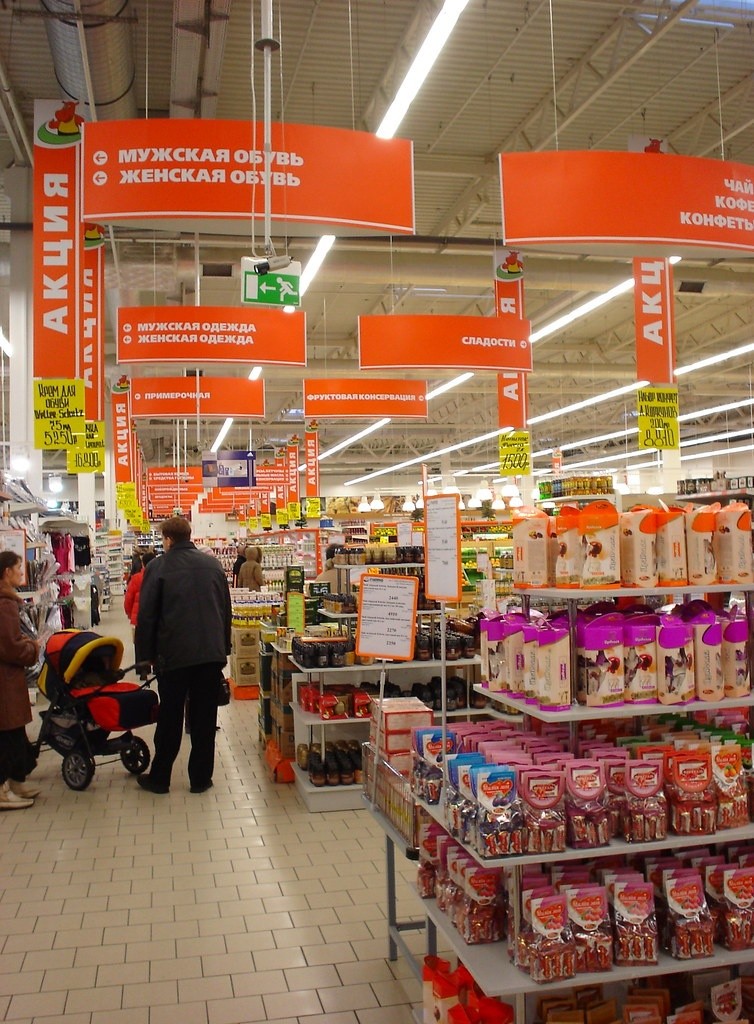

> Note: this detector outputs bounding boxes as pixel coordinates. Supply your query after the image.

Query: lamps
[358,359,556,513]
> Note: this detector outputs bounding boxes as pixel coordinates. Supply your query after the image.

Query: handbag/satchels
[218,671,229,707]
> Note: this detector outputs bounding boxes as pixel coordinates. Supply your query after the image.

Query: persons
[0,551,41,810]
[133,518,233,793]
[124,544,221,733]
[315,541,348,593]
[232,546,270,591]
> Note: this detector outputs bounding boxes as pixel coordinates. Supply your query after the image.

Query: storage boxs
[230,626,296,784]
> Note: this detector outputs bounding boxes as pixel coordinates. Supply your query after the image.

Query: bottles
[334,547,424,565]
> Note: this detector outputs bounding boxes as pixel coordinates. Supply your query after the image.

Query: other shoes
[0,782,34,809]
[191,779,213,794]
[9,779,39,799]
[137,775,169,794]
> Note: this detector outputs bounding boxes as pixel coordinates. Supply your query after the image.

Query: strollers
[28,628,156,792]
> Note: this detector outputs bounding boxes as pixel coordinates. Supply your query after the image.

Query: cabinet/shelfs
[0,501,126,703]
[287,488,754,1024]
